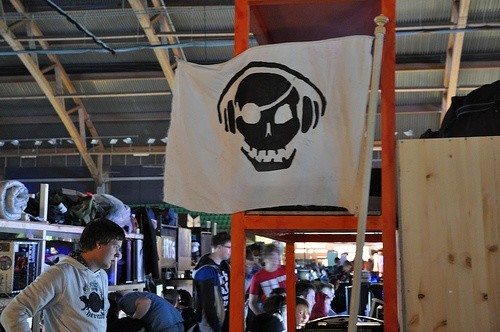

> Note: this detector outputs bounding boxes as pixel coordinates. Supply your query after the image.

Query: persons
[245,241,384,332]
[193,232,230,332]
[192,240,200,263]
[0,218,126,332]
[108,289,201,332]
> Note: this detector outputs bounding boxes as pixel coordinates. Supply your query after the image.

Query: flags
[161,34,374,216]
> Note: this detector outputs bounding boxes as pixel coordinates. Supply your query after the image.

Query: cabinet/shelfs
[0,189,145,332]
[154,223,213,309]
